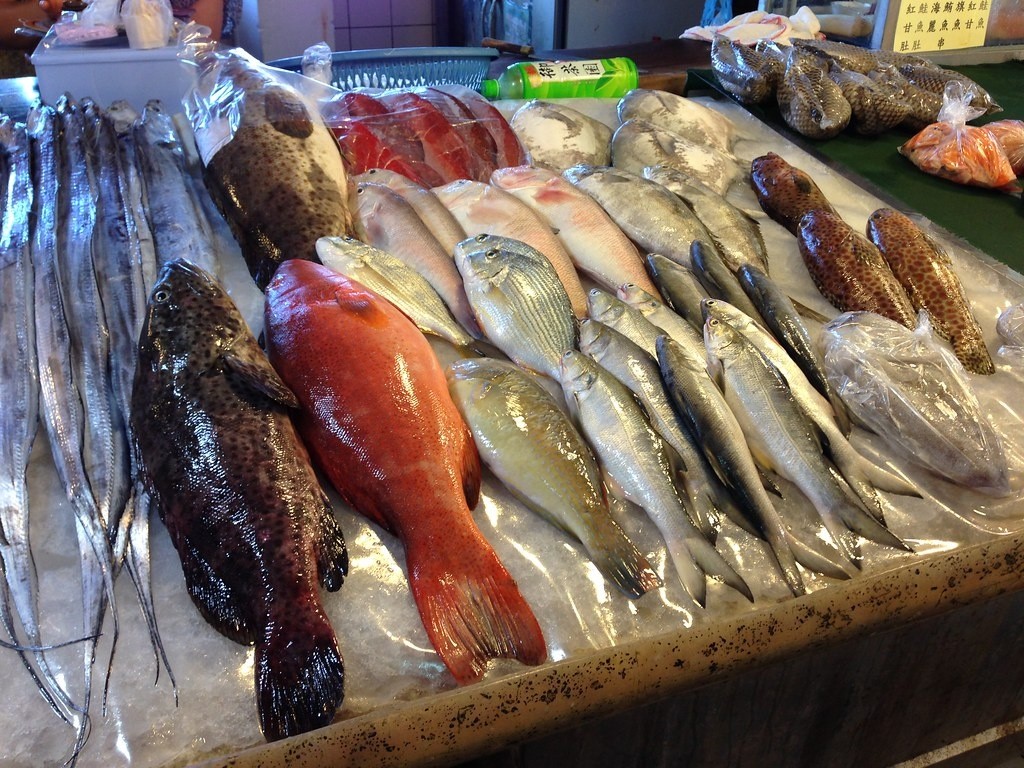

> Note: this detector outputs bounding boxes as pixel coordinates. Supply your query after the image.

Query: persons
[38,0,223,46]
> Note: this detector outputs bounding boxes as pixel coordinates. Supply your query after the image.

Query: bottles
[481,57,640,102]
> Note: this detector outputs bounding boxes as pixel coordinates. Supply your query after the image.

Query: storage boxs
[29,24,198,114]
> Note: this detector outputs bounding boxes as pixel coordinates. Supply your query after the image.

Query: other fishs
[128,259,351,745]
[1,89,222,768]
[211,29,1021,690]
